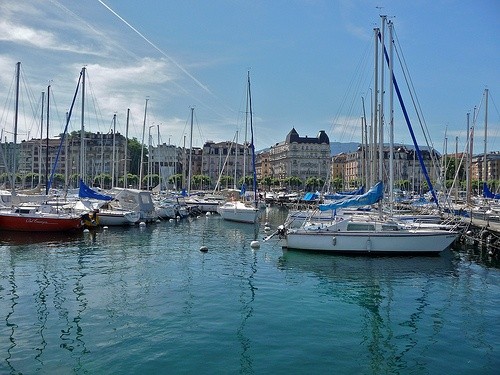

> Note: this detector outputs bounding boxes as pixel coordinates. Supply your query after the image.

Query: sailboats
[0,12,499,255]
